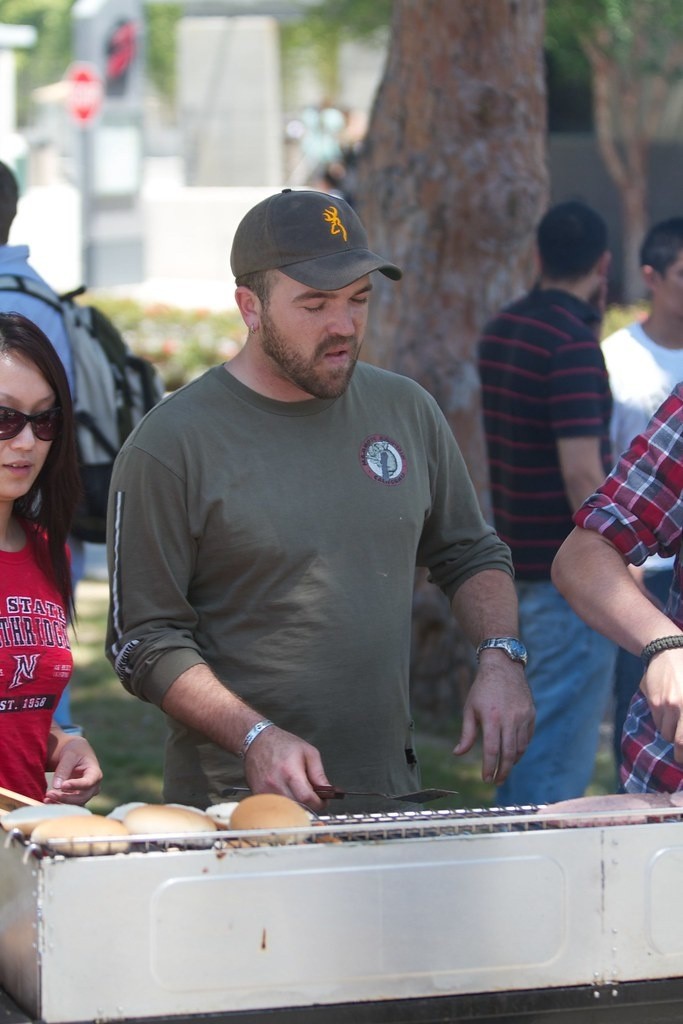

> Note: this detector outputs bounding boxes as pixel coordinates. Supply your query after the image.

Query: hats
[231,189,402,291]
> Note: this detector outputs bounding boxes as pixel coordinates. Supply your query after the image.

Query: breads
[0,793,311,857]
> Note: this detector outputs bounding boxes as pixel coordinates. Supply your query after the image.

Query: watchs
[476,637,527,670]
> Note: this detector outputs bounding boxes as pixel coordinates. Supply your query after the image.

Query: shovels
[309,784,457,806]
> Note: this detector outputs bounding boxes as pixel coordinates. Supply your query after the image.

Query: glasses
[0,405,62,439]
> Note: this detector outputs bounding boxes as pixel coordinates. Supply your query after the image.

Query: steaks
[531,791,683,827]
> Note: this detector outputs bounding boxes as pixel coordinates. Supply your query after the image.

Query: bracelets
[641,633,683,667]
[236,719,275,759]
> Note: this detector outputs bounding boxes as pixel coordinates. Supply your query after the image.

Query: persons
[0,164,683,817]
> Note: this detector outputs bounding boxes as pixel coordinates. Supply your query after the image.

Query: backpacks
[0,274,163,544]
